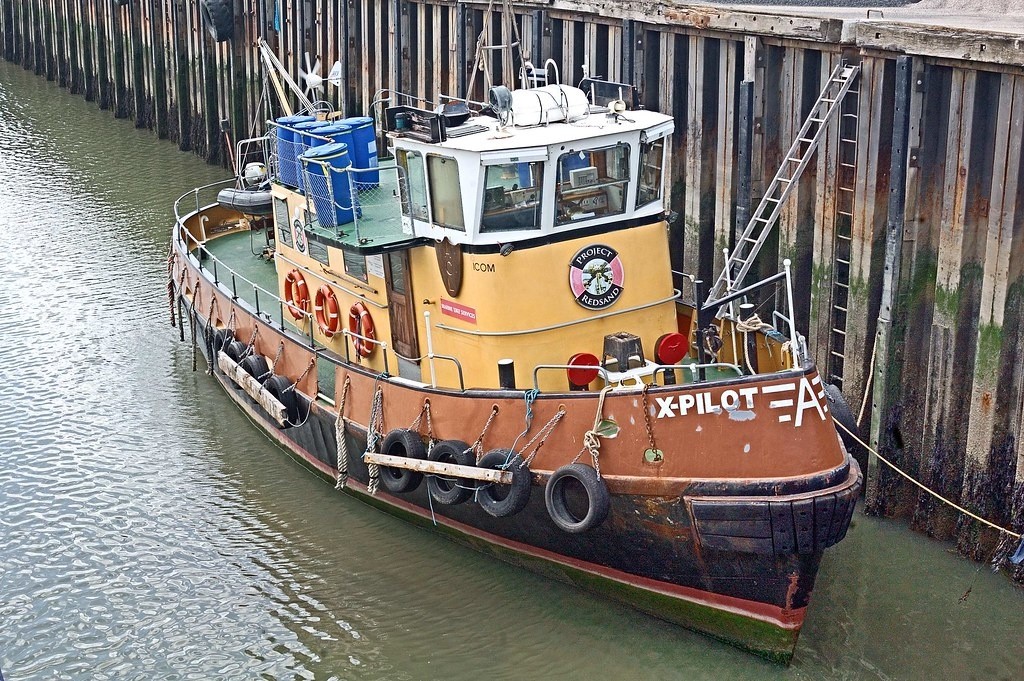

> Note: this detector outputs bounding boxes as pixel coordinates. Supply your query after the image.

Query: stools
[603,331,646,373]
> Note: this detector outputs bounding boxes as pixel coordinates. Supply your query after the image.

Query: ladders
[700,64,858,320]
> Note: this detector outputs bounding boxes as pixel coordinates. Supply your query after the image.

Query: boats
[167,59,863,667]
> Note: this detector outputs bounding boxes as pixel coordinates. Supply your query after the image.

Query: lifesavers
[543,463,611,536]
[284,270,308,321]
[425,439,477,505]
[347,301,376,358]
[378,427,426,494]
[314,284,339,338]
[473,448,533,520]
[213,328,299,430]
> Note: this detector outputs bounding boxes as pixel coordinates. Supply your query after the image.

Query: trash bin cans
[275,114,380,228]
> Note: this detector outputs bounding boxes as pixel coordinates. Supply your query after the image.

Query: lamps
[499,244,514,257]
[665,209,678,225]
